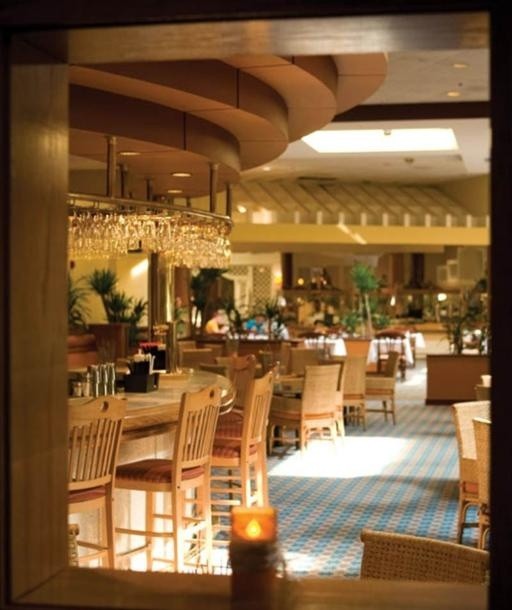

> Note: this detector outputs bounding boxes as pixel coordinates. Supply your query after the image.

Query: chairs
[199,362,231,378]
[366,352,401,424]
[452,400,491,543]
[182,350,212,369]
[474,384,490,400]
[211,371,274,546]
[323,359,345,440]
[214,365,278,509]
[375,331,406,380]
[70,398,127,570]
[205,344,221,359]
[481,374,492,384]
[114,384,223,572]
[473,418,492,547]
[343,356,366,431]
[229,355,257,409]
[288,348,321,377]
[272,365,341,455]
[178,341,196,365]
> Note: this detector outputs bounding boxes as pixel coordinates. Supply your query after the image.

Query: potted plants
[344,261,388,338]
[452,277,490,355]
[192,266,297,339]
[89,271,148,348]
[69,273,90,332]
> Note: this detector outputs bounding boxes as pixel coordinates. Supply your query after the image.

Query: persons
[246,312,266,335]
[206,306,230,334]
[227,308,241,336]
